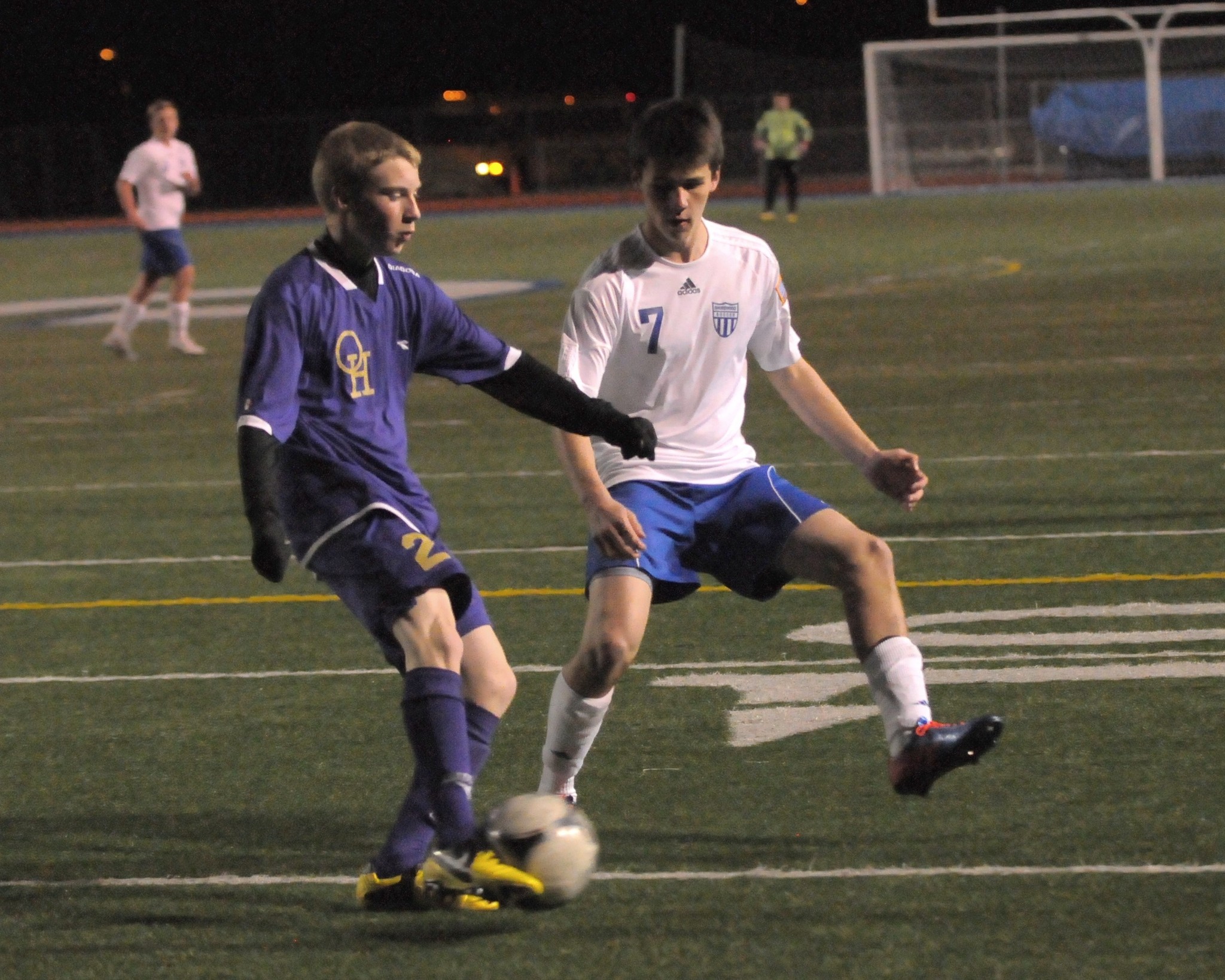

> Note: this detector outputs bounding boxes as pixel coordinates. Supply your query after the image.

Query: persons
[753,92,812,223]
[232,121,658,910]
[538,94,1003,801]
[103,100,206,357]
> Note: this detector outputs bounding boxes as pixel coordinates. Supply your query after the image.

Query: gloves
[238,425,287,582]
[474,351,657,461]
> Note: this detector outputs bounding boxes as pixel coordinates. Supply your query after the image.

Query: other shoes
[103,335,137,361]
[759,211,775,221]
[787,213,798,223]
[169,337,205,355]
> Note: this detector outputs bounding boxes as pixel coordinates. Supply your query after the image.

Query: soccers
[483,794,598,904]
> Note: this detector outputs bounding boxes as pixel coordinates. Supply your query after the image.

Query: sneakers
[412,825,545,900]
[888,715,1003,795]
[354,858,509,911]
